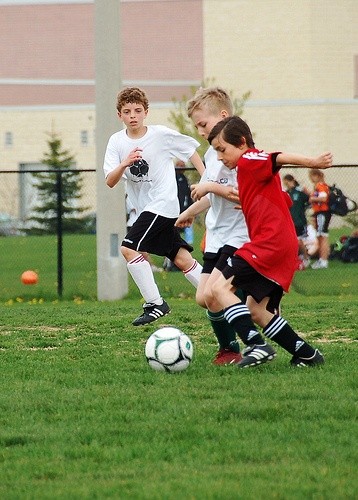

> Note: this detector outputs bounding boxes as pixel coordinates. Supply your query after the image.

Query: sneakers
[210,349,244,367]
[234,341,277,368]
[289,349,325,367]
[132,298,172,326]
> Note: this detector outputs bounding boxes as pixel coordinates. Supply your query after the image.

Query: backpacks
[328,184,348,216]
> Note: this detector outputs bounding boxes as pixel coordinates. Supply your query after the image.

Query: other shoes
[311,258,328,270]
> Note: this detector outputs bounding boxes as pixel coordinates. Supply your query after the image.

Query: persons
[283,174,311,271]
[339,230,358,263]
[101,87,206,327]
[328,236,349,260]
[301,215,318,257]
[208,116,335,368]
[173,87,252,367]
[300,168,333,269]
[162,158,194,271]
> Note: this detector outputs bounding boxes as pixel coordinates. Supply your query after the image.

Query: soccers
[20,269,39,285]
[144,326,194,375]
